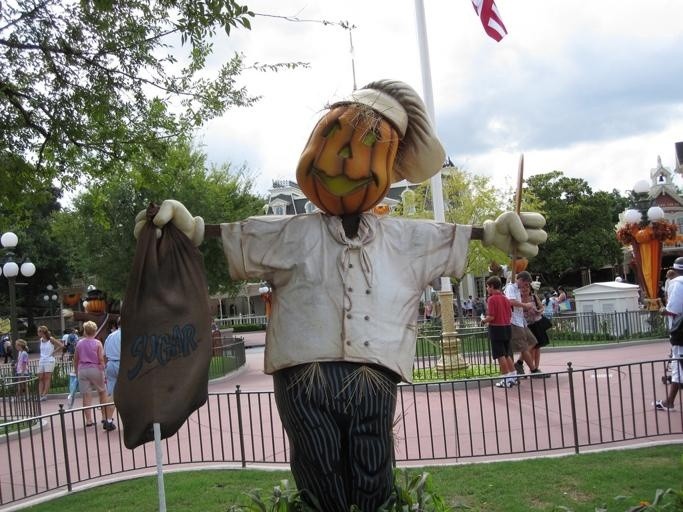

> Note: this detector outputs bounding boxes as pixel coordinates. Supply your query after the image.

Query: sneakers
[513,362,528,378]
[11,394,48,402]
[651,399,676,412]
[100,417,116,431]
[529,369,552,378]
[496,375,520,389]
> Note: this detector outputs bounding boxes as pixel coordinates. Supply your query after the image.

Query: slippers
[85,421,97,427]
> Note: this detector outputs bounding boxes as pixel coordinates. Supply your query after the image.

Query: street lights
[42,284,58,337]
[623,181,665,326]
[257,286,271,320]
[0,230,35,363]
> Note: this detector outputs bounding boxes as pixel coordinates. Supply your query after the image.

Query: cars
[452,298,473,316]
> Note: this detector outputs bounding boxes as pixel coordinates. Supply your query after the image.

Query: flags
[472,1,508,42]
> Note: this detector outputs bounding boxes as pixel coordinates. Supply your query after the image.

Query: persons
[486,275,520,387]
[424,286,568,319]
[506,270,551,378]
[2,317,122,432]
[520,283,549,373]
[132,79,549,512]
[650,257,683,412]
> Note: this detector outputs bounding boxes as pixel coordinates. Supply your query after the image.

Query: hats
[673,256,683,271]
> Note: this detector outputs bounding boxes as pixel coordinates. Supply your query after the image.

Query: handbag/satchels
[558,293,573,312]
[667,314,683,347]
[540,316,553,331]
